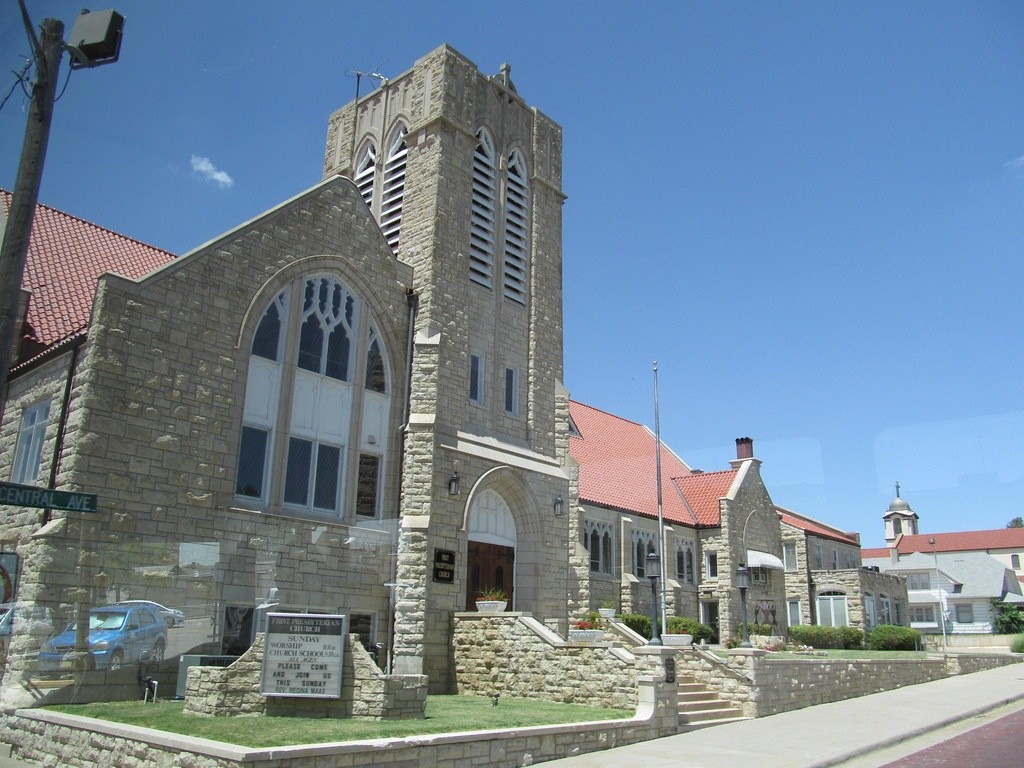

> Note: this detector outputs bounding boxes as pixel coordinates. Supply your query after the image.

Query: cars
[0,599,186,682]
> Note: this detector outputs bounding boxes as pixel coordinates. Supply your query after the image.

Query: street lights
[928,536,948,647]
[733,563,753,649]
[644,553,663,645]
